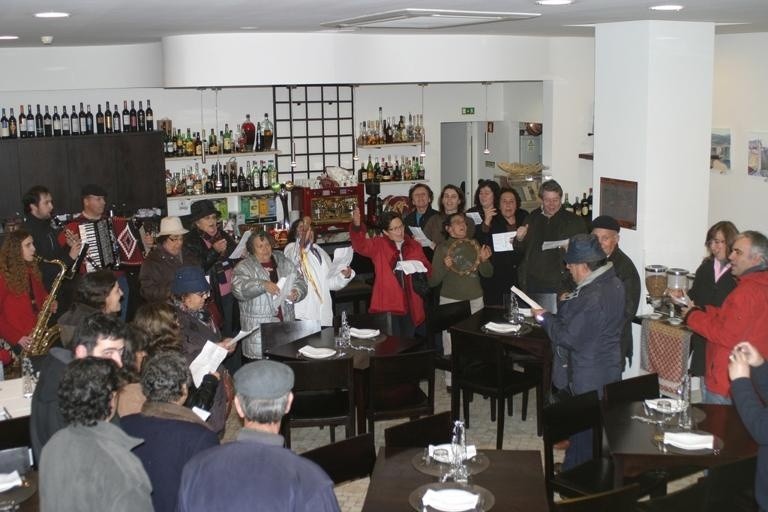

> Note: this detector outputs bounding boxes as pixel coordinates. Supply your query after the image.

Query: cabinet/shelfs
[165,147,280,226]
[356,137,428,184]
[68,131,168,219]
[0,136,68,218]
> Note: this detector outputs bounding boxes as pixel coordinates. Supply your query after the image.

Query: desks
[634,312,695,400]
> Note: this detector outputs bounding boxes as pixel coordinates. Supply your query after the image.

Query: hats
[153,216,190,241]
[80,183,108,197]
[186,199,222,225]
[591,214,620,233]
[563,232,608,264]
[169,265,214,298]
[232,360,296,400]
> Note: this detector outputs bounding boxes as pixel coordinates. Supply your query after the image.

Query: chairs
[536,372,668,512]
[0,415,33,475]
[260,280,557,487]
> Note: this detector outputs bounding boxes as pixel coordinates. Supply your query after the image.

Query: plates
[298,328,381,359]
[645,397,689,413]
[648,312,683,325]
[481,307,536,336]
[428,443,477,463]
[1,469,24,495]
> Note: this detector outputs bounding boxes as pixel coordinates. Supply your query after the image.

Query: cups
[295,178,321,190]
[656,400,672,426]
[337,174,357,188]
[432,449,450,472]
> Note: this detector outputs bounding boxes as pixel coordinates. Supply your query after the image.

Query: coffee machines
[363,177,384,220]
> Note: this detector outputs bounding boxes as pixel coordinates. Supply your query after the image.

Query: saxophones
[19,255,67,360]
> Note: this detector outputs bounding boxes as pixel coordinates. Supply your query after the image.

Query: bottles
[509,293,519,324]
[165,159,277,196]
[452,425,467,484]
[339,311,350,347]
[24,371,33,397]
[563,186,592,216]
[0,98,154,141]
[357,105,423,146]
[677,373,694,428]
[162,111,273,158]
[643,262,691,316]
[358,153,426,183]
